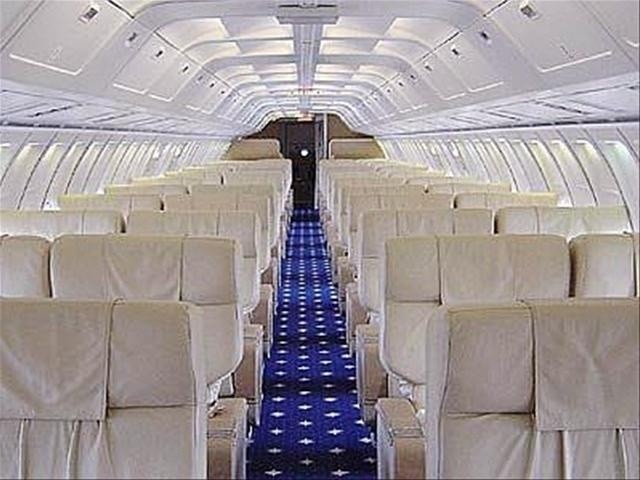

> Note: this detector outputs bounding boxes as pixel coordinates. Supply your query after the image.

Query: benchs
[2,233,248,478]
[2,294,209,480]
[2,160,294,353]
[373,232,640,480]
[425,296,640,480]
[316,159,628,347]
